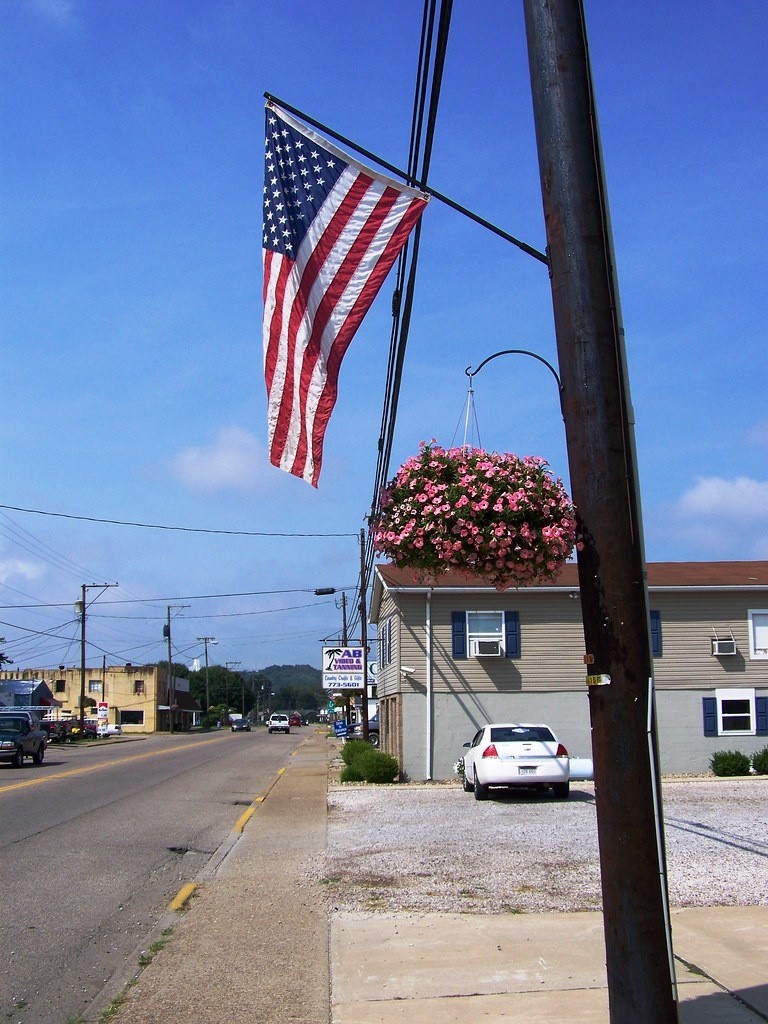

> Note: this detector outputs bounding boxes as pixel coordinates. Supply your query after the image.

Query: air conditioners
[712,639,737,656]
[470,638,501,657]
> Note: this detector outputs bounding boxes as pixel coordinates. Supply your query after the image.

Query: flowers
[373,438,585,597]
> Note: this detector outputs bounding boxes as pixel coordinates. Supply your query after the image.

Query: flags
[257,99,431,490]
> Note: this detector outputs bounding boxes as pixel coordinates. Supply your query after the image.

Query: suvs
[268,713,291,733]
[347,714,380,747]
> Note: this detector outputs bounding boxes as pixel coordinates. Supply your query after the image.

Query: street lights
[167,605,218,733]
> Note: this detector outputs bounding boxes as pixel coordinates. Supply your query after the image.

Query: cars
[0,716,45,767]
[0,705,122,749]
[231,719,251,732]
[461,724,570,801]
[266,716,309,727]
